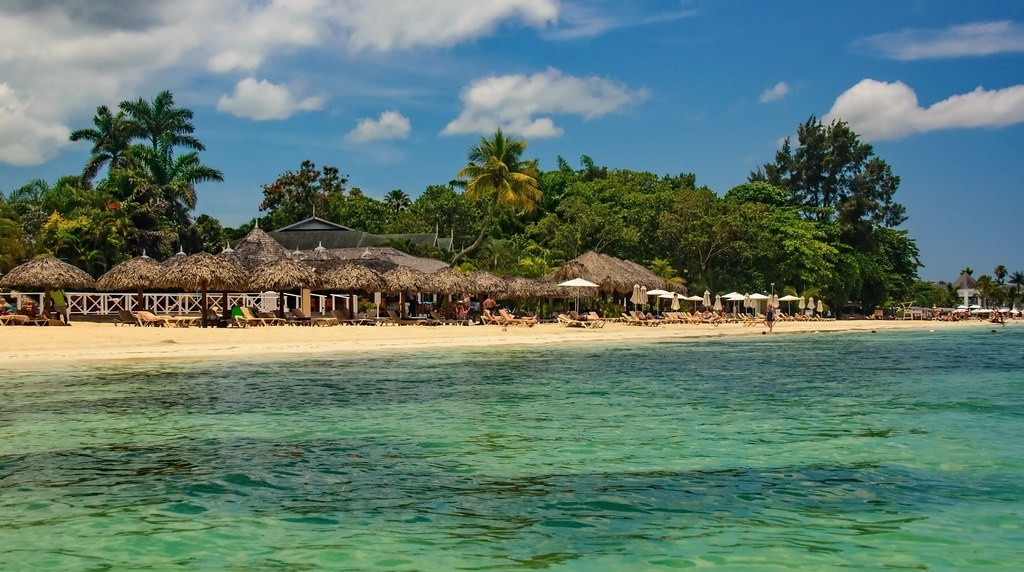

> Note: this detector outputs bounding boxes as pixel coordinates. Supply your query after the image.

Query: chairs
[0,307,836,328]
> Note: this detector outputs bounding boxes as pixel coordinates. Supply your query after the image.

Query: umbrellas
[639,286,648,312]
[671,293,703,312]
[95,218,603,328]
[0,253,95,309]
[569,251,688,316]
[558,278,600,314]
[647,289,668,319]
[702,289,711,310]
[712,295,722,313]
[817,300,823,317]
[953,304,1024,316]
[659,291,687,302]
[630,283,640,311]
[721,292,805,316]
[807,296,816,309]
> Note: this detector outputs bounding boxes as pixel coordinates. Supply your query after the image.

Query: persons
[766,304,776,332]
[232,301,252,320]
[49,287,69,324]
[0,298,14,312]
[483,294,501,313]
